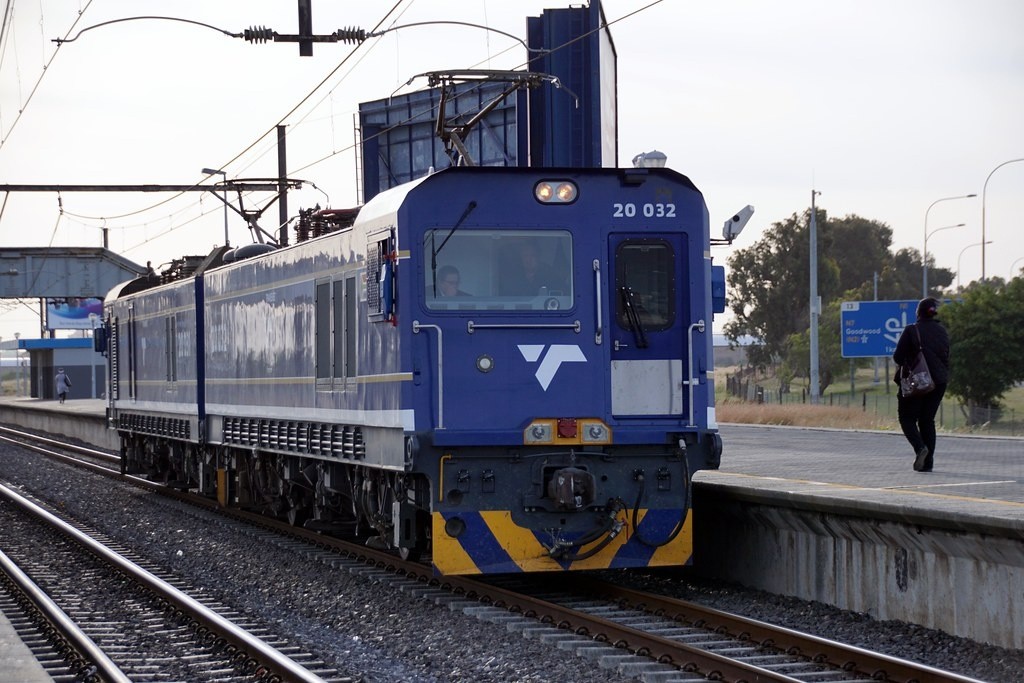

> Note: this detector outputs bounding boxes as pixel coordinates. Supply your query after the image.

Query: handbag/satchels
[900,350,934,396]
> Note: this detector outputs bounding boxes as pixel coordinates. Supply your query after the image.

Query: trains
[95,67,756,580]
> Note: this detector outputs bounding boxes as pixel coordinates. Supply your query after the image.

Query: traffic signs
[841,300,959,359]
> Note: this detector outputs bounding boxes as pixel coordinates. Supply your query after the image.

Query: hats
[916,297,940,316]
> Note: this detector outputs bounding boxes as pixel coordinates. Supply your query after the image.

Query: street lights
[923,193,979,301]
[957,240,992,293]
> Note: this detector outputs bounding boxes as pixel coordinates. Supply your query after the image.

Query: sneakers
[913,445,929,471]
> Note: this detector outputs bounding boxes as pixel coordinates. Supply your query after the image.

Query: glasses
[442,280,460,286]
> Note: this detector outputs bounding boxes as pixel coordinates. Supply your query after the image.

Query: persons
[496,241,563,297]
[429,264,476,311]
[54,368,69,404]
[892,297,950,472]
